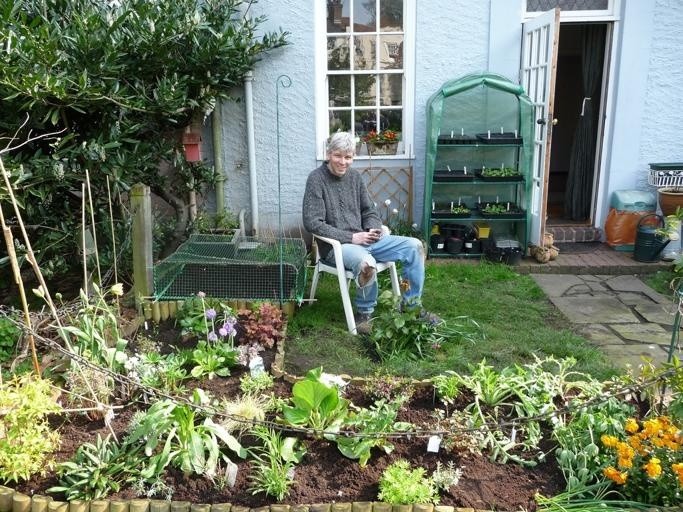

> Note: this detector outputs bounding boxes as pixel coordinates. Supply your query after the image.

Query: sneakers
[355,312,372,335]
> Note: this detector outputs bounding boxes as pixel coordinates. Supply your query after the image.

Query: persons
[303,131,445,334]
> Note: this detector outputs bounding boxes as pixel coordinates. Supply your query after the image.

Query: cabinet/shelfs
[426,72,535,259]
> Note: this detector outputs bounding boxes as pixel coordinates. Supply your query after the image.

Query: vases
[657,187,683,217]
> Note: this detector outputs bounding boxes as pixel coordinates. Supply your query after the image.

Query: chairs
[307,225,401,340]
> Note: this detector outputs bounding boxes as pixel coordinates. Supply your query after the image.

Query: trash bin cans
[607,191,657,252]
